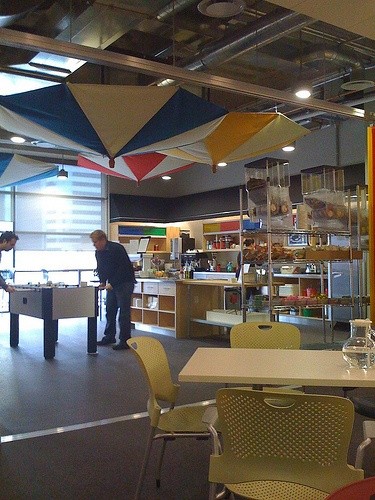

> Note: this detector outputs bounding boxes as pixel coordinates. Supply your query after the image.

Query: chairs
[126,321,375,500]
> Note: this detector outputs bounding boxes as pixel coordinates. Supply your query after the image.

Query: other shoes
[97,337,116,345]
[112,340,130,350]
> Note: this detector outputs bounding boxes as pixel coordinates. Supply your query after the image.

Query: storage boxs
[205,309,270,326]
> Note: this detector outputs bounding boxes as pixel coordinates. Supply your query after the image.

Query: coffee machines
[169,238,195,272]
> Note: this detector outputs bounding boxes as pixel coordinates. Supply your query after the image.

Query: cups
[132,298,142,307]
[154,245,159,250]
[80,281,87,287]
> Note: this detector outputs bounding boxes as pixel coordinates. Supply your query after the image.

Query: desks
[178,347,375,391]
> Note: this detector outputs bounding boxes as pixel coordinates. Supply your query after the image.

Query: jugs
[341,319,375,369]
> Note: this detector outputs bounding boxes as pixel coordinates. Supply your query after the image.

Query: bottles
[205,234,233,250]
[180,257,234,279]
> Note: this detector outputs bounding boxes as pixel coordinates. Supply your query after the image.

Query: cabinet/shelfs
[201,220,240,252]
[182,278,285,337]
[239,181,370,350]
[109,222,191,254]
[130,277,224,339]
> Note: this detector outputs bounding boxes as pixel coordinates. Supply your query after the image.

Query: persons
[0,231,18,294]
[235,239,262,313]
[90,230,133,350]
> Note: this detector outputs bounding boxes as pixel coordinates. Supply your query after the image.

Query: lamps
[282,139,296,153]
[293,29,311,99]
[56,153,68,180]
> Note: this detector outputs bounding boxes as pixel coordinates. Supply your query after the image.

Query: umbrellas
[0,83,311,188]
